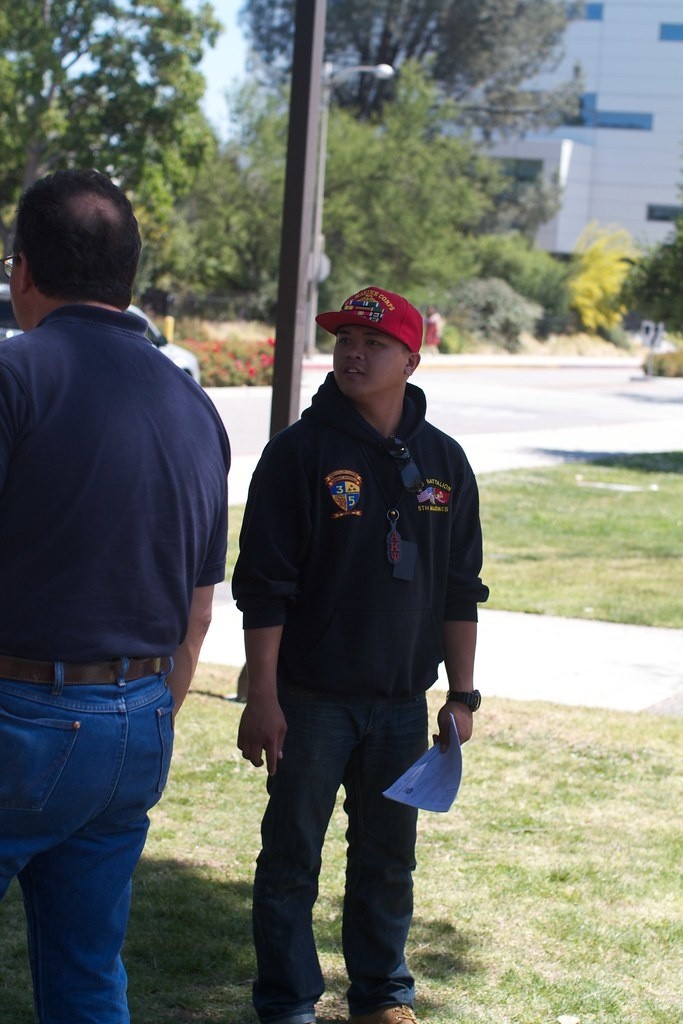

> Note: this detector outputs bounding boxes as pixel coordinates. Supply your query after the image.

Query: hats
[316,287,423,353]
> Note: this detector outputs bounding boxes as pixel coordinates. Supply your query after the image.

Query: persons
[0,164,232,1024]
[232,285,488,1024]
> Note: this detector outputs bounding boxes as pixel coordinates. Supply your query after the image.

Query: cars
[0,282,202,397]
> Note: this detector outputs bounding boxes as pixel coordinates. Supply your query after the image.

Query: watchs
[446,689,481,712]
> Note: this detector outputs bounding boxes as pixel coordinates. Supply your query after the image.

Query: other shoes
[349,1004,421,1024]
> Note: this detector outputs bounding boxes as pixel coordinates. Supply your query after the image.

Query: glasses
[0,255,19,278]
[383,434,422,493]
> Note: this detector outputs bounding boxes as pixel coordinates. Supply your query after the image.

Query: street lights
[301,63,393,363]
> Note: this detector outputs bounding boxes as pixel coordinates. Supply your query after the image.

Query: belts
[0,655,161,685]
